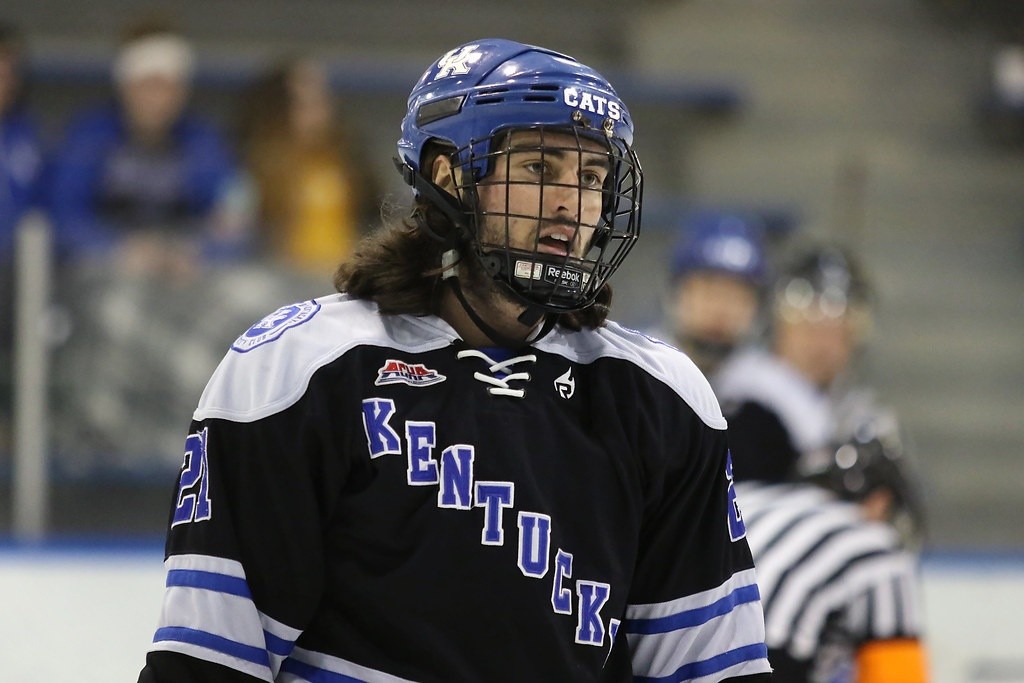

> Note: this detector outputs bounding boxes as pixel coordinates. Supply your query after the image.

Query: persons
[1,22,383,487]
[137,38,774,683]
[644,205,931,682]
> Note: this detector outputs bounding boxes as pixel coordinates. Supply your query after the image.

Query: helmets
[395,37,641,313]
[674,209,767,290]
